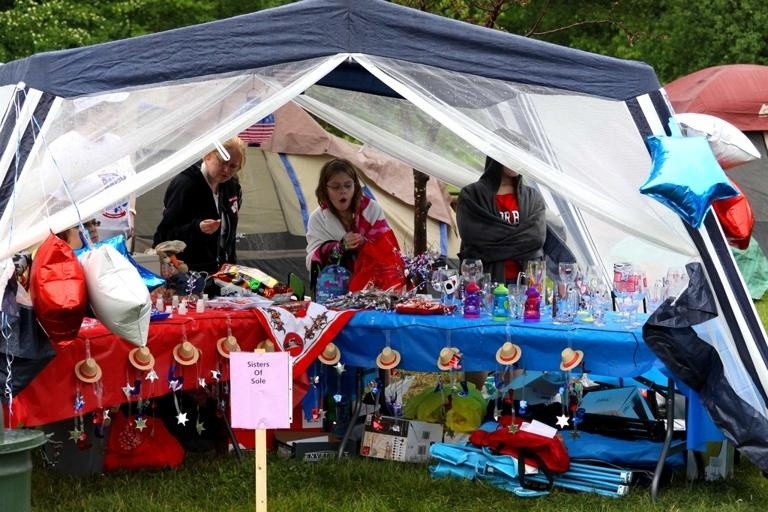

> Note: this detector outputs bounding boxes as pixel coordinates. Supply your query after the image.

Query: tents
[0,0,768,473]
[110,73,462,289]
[662,63,768,300]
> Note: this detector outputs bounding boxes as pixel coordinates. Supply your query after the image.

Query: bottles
[155,294,209,318]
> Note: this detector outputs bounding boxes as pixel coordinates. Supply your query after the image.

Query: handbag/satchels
[316,239,352,303]
[468,413,571,491]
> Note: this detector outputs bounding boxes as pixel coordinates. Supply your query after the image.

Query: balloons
[29,231,168,348]
[639,112,762,250]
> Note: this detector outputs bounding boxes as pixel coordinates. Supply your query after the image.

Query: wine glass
[432,259,688,327]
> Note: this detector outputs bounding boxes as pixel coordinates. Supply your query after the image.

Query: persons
[306,157,405,291]
[40,90,136,249]
[151,134,246,275]
[455,128,546,393]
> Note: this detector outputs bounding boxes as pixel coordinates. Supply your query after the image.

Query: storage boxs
[354,411,445,463]
[576,386,658,422]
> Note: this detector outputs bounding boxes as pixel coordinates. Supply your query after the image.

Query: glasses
[324,182,356,190]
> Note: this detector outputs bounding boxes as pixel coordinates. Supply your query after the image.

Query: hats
[318,342,342,366]
[437,346,462,371]
[559,347,585,371]
[375,347,402,370]
[216,336,240,359]
[129,345,155,371]
[74,358,102,384]
[495,341,522,366]
[172,341,199,365]
[256,339,275,352]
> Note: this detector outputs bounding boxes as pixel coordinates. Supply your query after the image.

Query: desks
[304,297,726,503]
[0,296,312,467]
